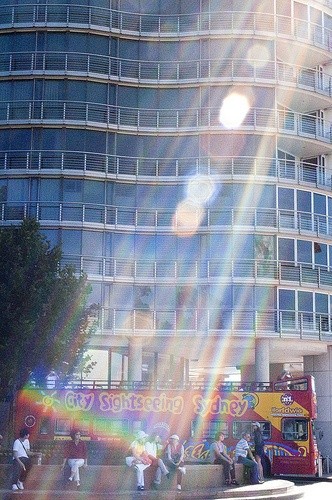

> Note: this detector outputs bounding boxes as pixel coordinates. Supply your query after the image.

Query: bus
[11,375,319,478]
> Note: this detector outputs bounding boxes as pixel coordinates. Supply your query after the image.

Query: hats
[169,435,180,440]
[136,431,148,440]
[252,422,260,427]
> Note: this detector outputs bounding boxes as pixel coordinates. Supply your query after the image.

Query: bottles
[38,456,41,465]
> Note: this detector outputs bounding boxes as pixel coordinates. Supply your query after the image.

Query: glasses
[73,434,81,436]
[246,436,252,438]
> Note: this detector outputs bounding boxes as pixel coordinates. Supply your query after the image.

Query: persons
[135,376,187,389]
[59,430,88,486]
[144,434,170,489]
[206,381,296,391]
[297,431,305,439]
[12,429,34,491]
[119,380,127,389]
[210,432,239,485]
[251,422,264,482]
[126,431,152,490]
[165,434,186,490]
[236,432,264,483]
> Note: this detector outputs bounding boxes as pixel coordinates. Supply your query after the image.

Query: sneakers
[17,479,24,490]
[12,484,18,490]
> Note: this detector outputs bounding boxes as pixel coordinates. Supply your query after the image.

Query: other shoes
[225,481,232,485]
[168,472,173,479]
[131,465,138,473]
[69,477,73,481]
[180,467,186,474]
[177,485,182,493]
[138,486,145,491]
[231,480,239,485]
[151,483,159,490]
[77,481,80,487]
